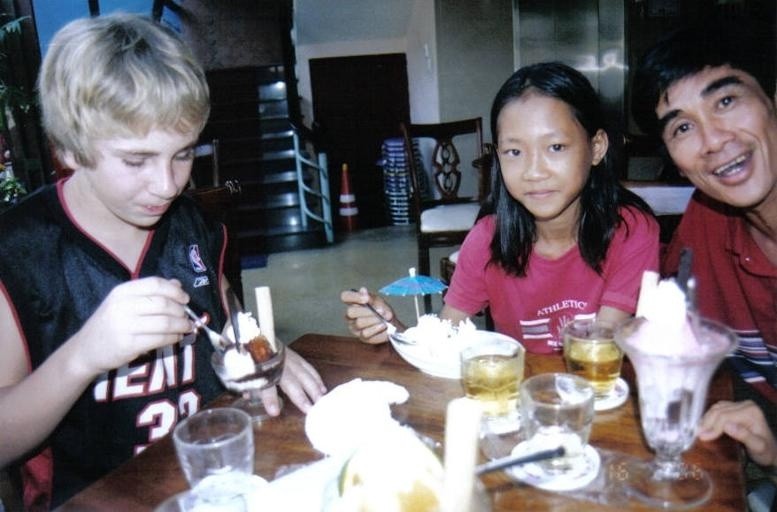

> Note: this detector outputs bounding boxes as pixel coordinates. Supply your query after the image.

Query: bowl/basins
[388,326,524,379]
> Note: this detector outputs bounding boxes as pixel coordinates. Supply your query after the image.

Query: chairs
[396,115,501,332]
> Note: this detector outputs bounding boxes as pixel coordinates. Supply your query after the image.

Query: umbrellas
[377,267,450,325]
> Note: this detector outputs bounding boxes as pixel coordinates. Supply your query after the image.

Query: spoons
[185,307,233,356]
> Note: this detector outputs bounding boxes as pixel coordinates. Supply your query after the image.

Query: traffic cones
[337,162,367,236]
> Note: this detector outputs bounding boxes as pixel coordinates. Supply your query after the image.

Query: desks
[52,330,751,511]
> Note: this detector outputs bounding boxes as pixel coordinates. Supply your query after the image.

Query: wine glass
[209,336,285,421]
[613,314,738,510]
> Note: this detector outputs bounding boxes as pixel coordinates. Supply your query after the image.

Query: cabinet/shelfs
[201,63,336,255]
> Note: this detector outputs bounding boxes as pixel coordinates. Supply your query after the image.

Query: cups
[517,372,595,486]
[174,406,254,492]
[459,343,527,435]
[563,318,624,401]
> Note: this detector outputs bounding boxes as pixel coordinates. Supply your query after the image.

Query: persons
[629,26,776,510]
[0,10,329,511]
[337,60,662,347]
[698,398,776,468]
